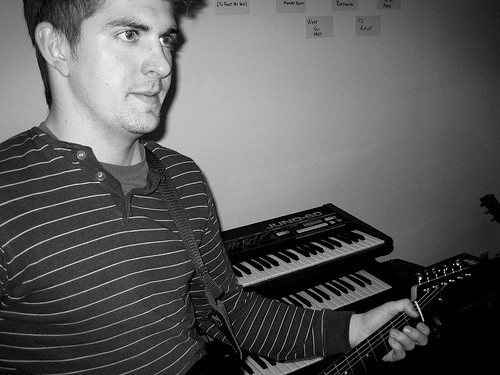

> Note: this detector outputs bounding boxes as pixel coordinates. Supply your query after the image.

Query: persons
[0,0,431,375]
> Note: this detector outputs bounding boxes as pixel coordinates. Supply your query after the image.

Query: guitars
[184,258,491,375]
[479,193,500,224]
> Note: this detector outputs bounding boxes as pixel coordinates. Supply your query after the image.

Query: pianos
[240,354,324,375]
[274,257,426,314]
[221,202,395,289]
